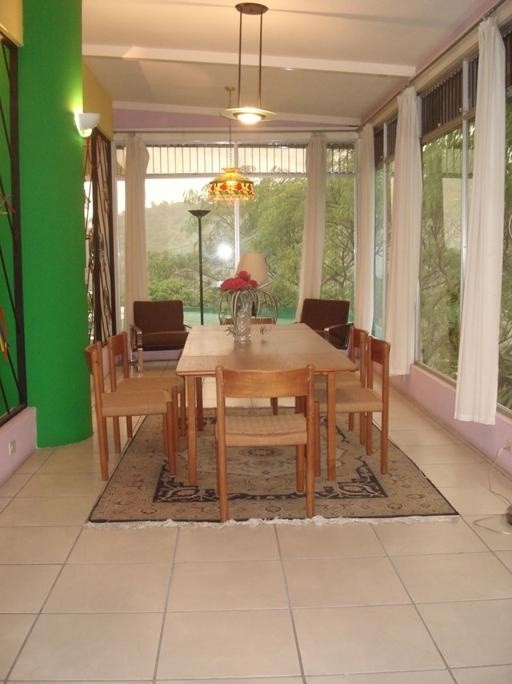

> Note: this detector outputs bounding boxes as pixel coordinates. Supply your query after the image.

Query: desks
[175,324,358,484]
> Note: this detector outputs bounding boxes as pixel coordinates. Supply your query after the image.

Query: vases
[231,291,253,342]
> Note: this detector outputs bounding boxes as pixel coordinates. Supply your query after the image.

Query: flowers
[217,271,278,336]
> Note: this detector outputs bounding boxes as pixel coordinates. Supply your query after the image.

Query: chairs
[107,332,185,457]
[301,298,354,350]
[314,336,390,481]
[295,325,369,445]
[215,364,315,522]
[85,341,177,481]
[129,300,192,373]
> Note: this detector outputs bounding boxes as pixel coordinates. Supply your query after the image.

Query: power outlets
[504,440,512,451]
[9,440,17,455]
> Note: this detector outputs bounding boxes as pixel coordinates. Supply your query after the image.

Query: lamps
[235,253,269,316]
[207,86,256,202]
[220,3,277,125]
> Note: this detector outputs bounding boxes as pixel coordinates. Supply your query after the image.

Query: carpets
[86,407,461,523]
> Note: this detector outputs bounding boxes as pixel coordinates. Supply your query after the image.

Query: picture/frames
[92,127,116,346]
